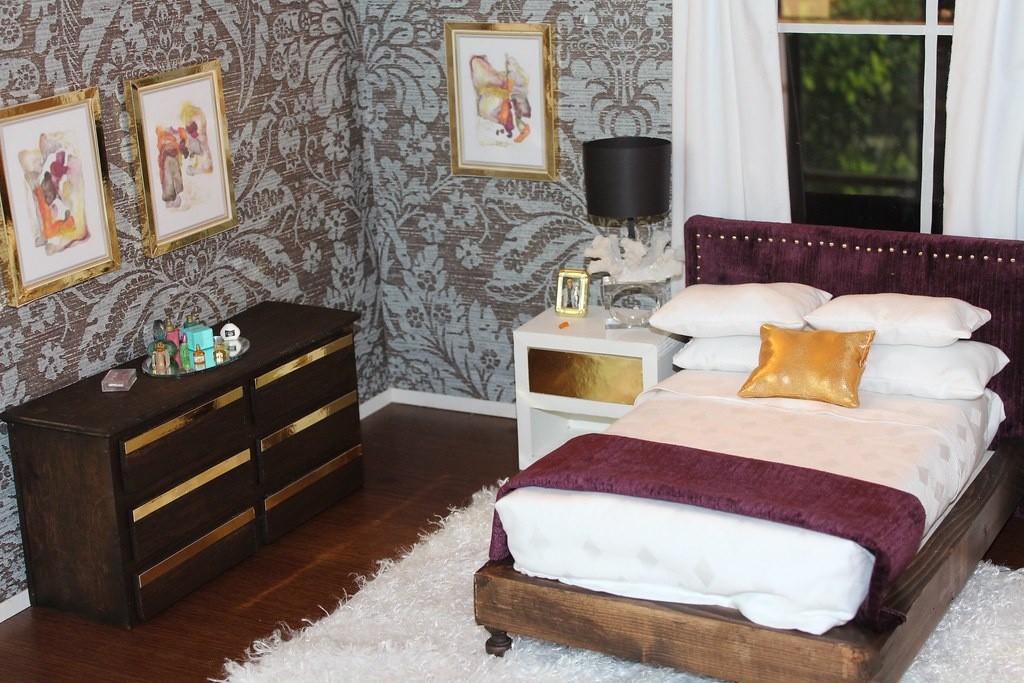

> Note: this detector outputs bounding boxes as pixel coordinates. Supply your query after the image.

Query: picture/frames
[123,59,240,261]
[555,270,590,317]
[444,21,559,182]
[0,87,122,310]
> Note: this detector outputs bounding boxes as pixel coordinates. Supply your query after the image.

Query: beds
[475,212,1024,683]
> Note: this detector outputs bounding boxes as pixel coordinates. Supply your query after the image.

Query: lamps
[583,136,673,328]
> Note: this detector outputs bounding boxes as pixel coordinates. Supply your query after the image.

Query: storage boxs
[182,324,214,351]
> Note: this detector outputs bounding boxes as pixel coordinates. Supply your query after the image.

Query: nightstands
[513,303,689,471]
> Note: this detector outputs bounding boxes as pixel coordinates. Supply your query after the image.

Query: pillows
[648,283,834,338]
[672,337,763,370]
[737,323,877,409]
[803,292,992,348]
[859,341,1012,401]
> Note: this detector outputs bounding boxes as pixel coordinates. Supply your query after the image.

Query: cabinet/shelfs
[0,300,367,631]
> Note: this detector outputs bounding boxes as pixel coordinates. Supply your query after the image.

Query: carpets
[208,477,1024,683]
